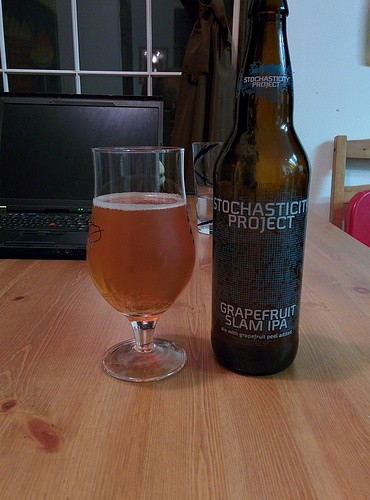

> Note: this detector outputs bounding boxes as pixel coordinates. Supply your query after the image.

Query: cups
[196,193,213,234]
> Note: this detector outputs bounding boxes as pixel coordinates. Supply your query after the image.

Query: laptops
[0,92,166,260]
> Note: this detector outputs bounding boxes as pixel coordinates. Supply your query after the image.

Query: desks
[2,190,368,499]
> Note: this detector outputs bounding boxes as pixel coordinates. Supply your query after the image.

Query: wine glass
[86,146,196,383]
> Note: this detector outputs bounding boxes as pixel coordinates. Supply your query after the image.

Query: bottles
[211,0,311,375]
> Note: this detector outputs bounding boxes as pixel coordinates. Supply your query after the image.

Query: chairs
[329,135,370,243]
